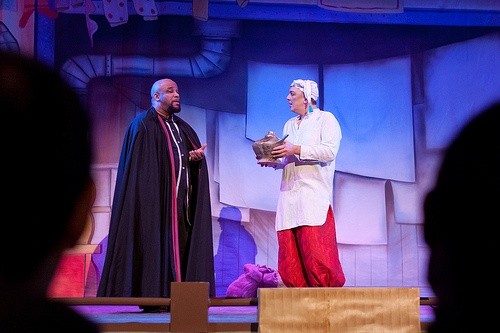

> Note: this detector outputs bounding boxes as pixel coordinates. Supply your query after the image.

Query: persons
[94,77,217,312]
[420,97,500,332]
[263,79,349,288]
[1,47,107,331]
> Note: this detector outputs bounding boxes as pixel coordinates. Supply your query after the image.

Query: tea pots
[251,130,289,164]
